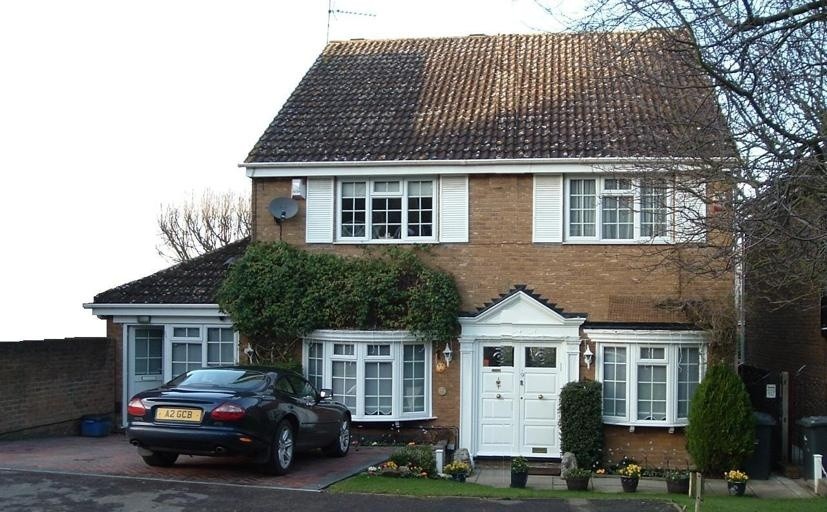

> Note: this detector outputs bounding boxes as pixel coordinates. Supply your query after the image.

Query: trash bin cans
[798,415,827,481]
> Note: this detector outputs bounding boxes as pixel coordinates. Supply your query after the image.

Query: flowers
[724,470,748,483]
[621,463,642,478]
[443,462,470,475]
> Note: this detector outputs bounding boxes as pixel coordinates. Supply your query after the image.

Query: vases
[728,482,747,496]
[621,478,639,493]
[453,475,467,482]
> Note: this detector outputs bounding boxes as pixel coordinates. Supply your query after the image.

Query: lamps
[442,342,452,367]
[583,344,593,370]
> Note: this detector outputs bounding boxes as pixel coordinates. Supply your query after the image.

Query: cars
[125,364,352,474]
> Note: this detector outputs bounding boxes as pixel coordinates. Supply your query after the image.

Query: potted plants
[563,468,592,491]
[510,455,528,487]
[664,469,690,494]
